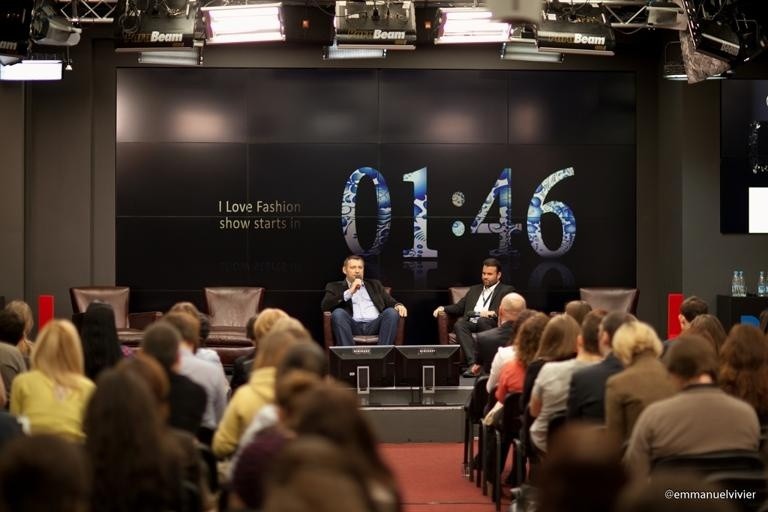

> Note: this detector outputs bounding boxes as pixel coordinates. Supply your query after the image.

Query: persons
[0,300,403,512]
[321,255,408,387]
[433,258,515,378]
[472,292,768,512]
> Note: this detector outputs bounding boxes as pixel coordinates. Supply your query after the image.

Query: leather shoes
[463,364,481,376]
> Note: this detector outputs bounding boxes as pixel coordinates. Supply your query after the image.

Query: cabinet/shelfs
[715,292,768,333]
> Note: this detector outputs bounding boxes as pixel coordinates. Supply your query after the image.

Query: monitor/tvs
[328,345,395,387]
[394,344,461,386]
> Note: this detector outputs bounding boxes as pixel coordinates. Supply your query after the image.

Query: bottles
[732,271,748,298]
[755,271,768,297]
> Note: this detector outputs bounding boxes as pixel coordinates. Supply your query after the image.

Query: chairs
[549,287,641,345]
[320,285,407,395]
[187,283,265,380]
[68,285,165,356]
[462,373,582,511]
[437,284,518,369]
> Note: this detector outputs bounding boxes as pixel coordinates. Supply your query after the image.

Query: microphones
[467,311,497,318]
[355,276,362,290]
